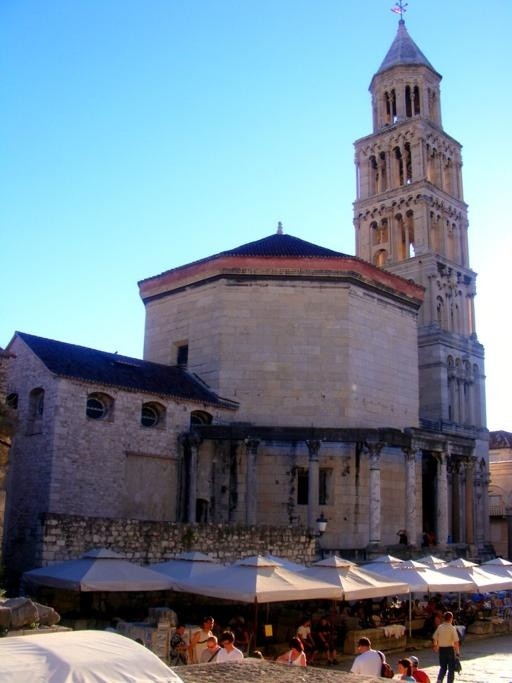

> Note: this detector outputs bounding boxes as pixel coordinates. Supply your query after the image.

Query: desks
[344,624,408,654]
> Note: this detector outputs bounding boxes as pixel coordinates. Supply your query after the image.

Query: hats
[357,638,371,646]
[409,656,418,663]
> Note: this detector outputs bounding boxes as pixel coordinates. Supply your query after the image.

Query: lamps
[306,512,328,538]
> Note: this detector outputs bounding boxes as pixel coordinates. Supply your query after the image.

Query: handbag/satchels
[452,659,461,671]
[381,663,394,678]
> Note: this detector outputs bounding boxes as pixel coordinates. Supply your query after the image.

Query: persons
[164,589,511,683]
